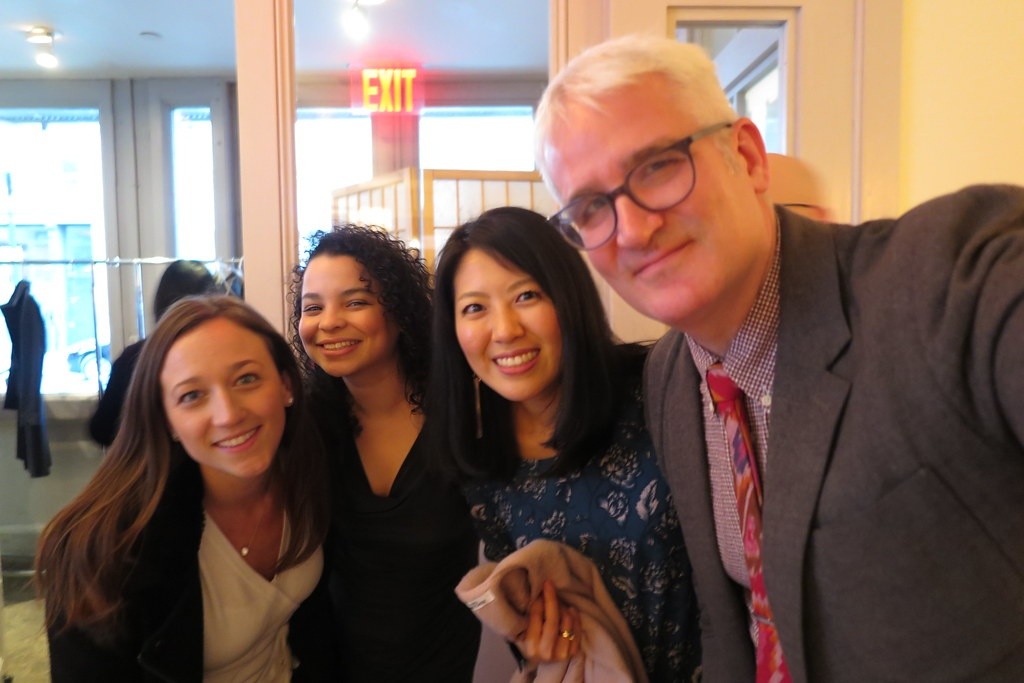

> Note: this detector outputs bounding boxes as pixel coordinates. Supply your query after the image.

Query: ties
[706,363,793,683]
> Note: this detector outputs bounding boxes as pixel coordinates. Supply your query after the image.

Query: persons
[288,225,474,683]
[37,296,326,683]
[89,260,216,446]
[767,150,830,223]
[432,207,698,683]
[536,38,1024,683]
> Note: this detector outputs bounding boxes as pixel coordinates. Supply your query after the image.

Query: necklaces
[227,512,263,557]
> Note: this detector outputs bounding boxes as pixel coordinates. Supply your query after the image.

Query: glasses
[546,123,732,249]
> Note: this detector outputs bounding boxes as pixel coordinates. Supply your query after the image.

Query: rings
[559,628,576,641]
